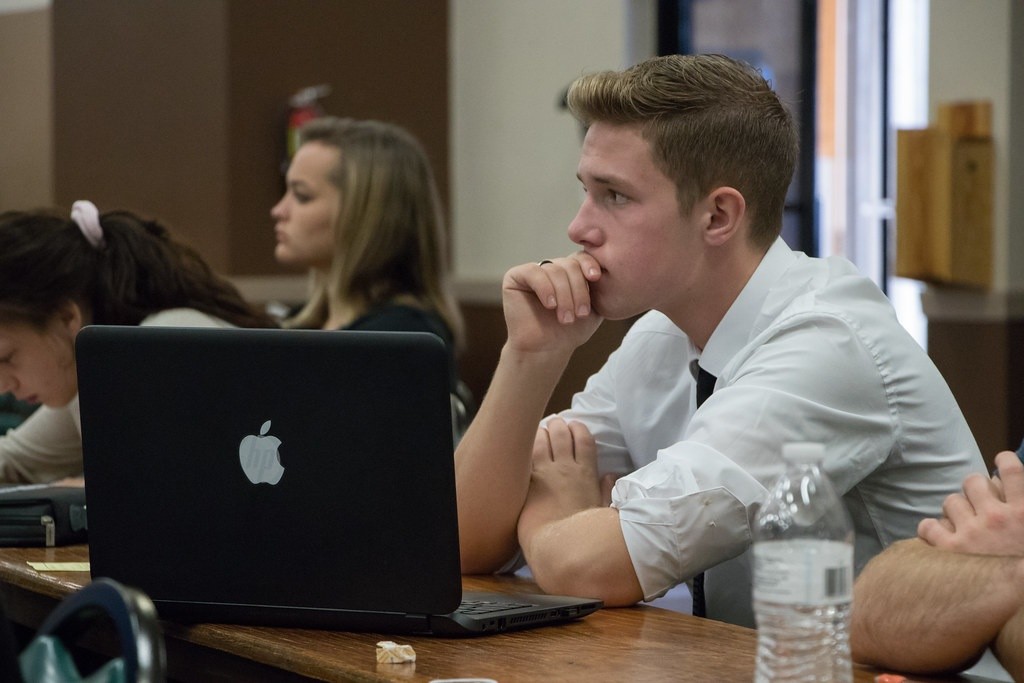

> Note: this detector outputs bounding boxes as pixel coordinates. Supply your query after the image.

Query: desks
[0,543,1003,683]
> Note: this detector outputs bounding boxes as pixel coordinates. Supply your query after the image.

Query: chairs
[14,578,168,683]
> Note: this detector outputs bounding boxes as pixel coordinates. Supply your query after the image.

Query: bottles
[749,438,857,683]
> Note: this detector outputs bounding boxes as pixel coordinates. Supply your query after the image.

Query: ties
[692,366,717,618]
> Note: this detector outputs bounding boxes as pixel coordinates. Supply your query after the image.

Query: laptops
[77,325,605,641]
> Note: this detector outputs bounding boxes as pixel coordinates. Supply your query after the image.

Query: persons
[269,114,465,401]
[845,436,1023,682]
[454,51,996,609]
[1,199,285,489]
